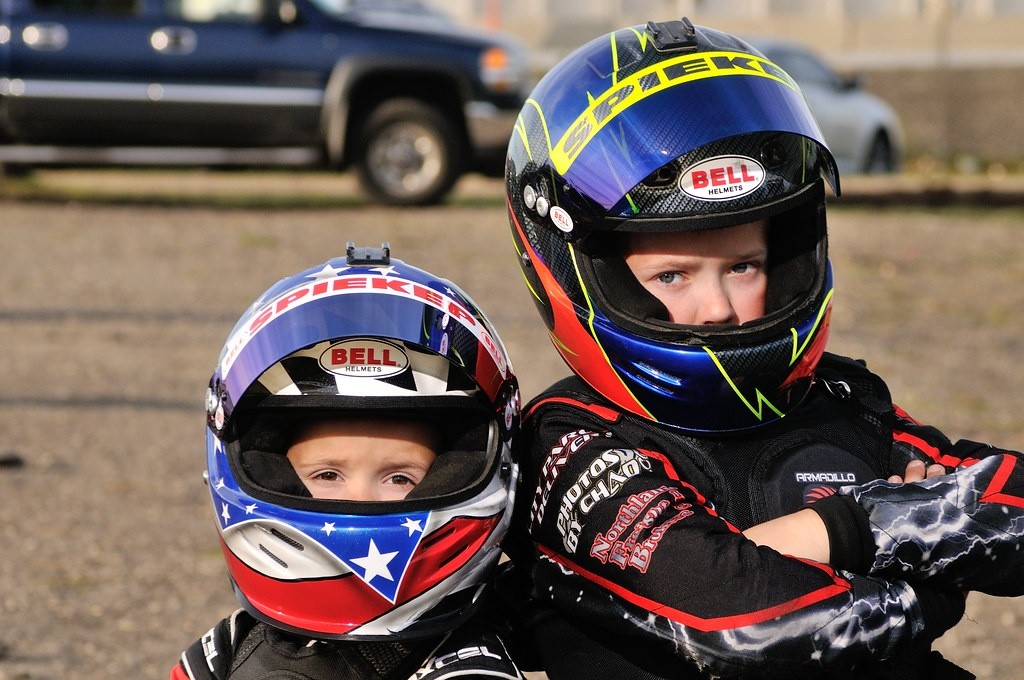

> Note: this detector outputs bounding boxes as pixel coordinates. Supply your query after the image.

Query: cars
[757,43,908,179]
[0,0,536,209]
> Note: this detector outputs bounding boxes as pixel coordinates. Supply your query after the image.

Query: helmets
[506,20,840,431]
[201,244,517,641]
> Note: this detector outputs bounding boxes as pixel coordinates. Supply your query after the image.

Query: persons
[170,242,527,680]
[497,18,1024,680]
